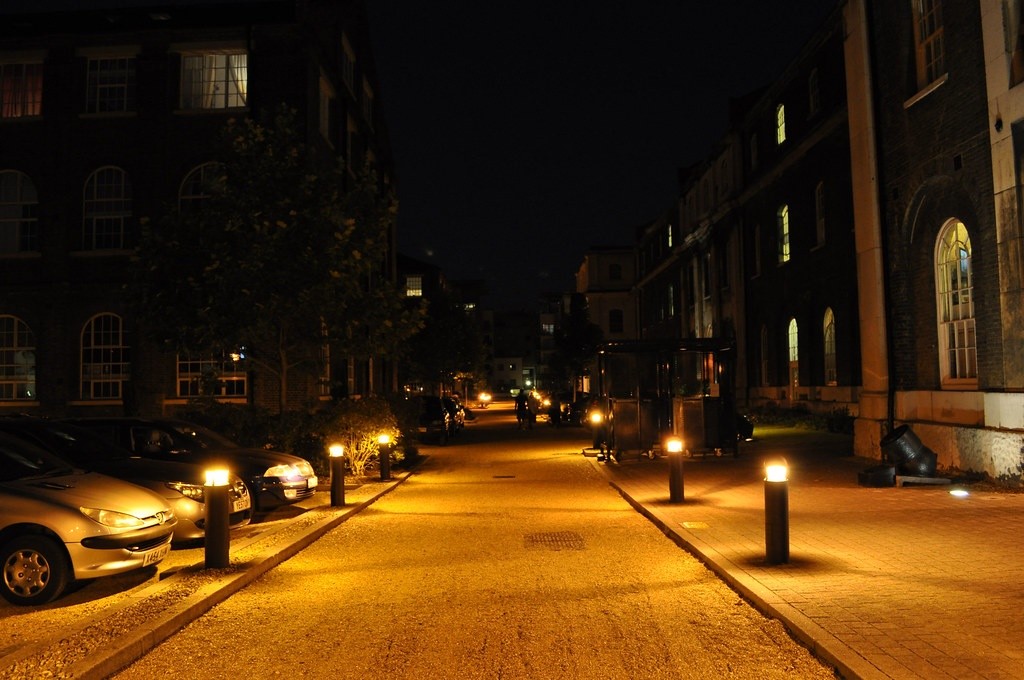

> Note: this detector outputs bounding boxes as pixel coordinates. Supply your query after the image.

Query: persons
[515,388,537,432]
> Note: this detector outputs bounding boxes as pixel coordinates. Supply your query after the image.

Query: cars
[0,412,179,605]
[44,413,252,545]
[170,419,318,509]
[415,394,465,446]
